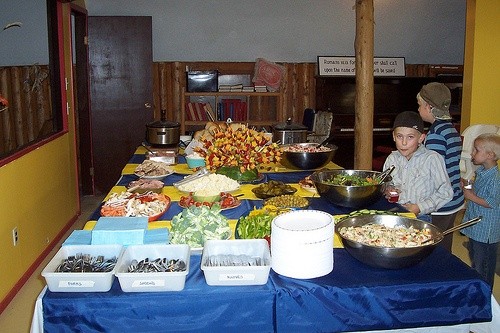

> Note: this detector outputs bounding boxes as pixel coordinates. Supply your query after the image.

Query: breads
[194,122,238,137]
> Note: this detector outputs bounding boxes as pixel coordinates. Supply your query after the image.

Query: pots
[269,117,308,145]
[145,117,180,146]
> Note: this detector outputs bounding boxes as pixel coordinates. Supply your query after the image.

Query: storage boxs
[41,245,125,293]
[200,240,272,286]
[115,244,191,291]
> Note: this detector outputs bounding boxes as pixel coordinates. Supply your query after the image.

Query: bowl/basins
[334,214,445,270]
[281,142,339,170]
[261,198,310,209]
[184,156,205,169]
[310,169,393,209]
[127,180,164,194]
[251,187,297,199]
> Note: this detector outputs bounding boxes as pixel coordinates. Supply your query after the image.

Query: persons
[417,82,464,253]
[383,111,454,224]
[460,133,500,293]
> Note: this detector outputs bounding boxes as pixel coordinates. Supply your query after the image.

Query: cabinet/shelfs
[181,86,283,136]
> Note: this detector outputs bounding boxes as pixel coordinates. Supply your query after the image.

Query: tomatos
[179,192,241,209]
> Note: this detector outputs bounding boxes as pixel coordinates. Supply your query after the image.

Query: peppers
[236,204,277,239]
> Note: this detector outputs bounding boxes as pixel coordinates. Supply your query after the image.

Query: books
[185,99,246,122]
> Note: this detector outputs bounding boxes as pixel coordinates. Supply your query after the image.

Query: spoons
[127,257,185,273]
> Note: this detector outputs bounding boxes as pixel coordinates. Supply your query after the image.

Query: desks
[29,142,500,333]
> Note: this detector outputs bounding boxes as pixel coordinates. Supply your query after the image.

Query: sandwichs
[173,168,240,192]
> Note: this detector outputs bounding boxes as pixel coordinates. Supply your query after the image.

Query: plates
[100,194,171,222]
[190,235,231,255]
[178,199,241,210]
[271,209,333,279]
[235,209,252,240]
[134,169,175,180]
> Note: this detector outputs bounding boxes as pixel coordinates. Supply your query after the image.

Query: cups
[387,182,402,202]
[463,179,473,201]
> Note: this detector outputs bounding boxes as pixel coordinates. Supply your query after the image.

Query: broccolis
[169,202,230,249]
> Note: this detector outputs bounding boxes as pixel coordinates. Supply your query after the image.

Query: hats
[420,82,451,111]
[393,111,424,133]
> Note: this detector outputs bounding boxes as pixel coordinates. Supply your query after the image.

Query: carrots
[100,206,125,217]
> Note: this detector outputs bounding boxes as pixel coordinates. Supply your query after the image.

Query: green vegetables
[319,173,381,187]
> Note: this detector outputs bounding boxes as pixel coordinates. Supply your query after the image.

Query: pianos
[313,74,437,140]
